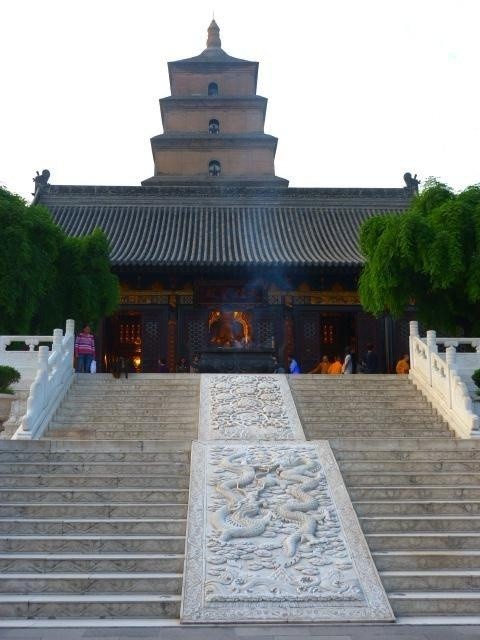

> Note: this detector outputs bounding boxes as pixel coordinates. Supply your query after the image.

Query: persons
[210,311,248,346]
[75,324,96,373]
[308,344,377,374]
[396,353,410,374]
[287,352,301,374]
[158,360,169,373]
[176,357,189,373]
